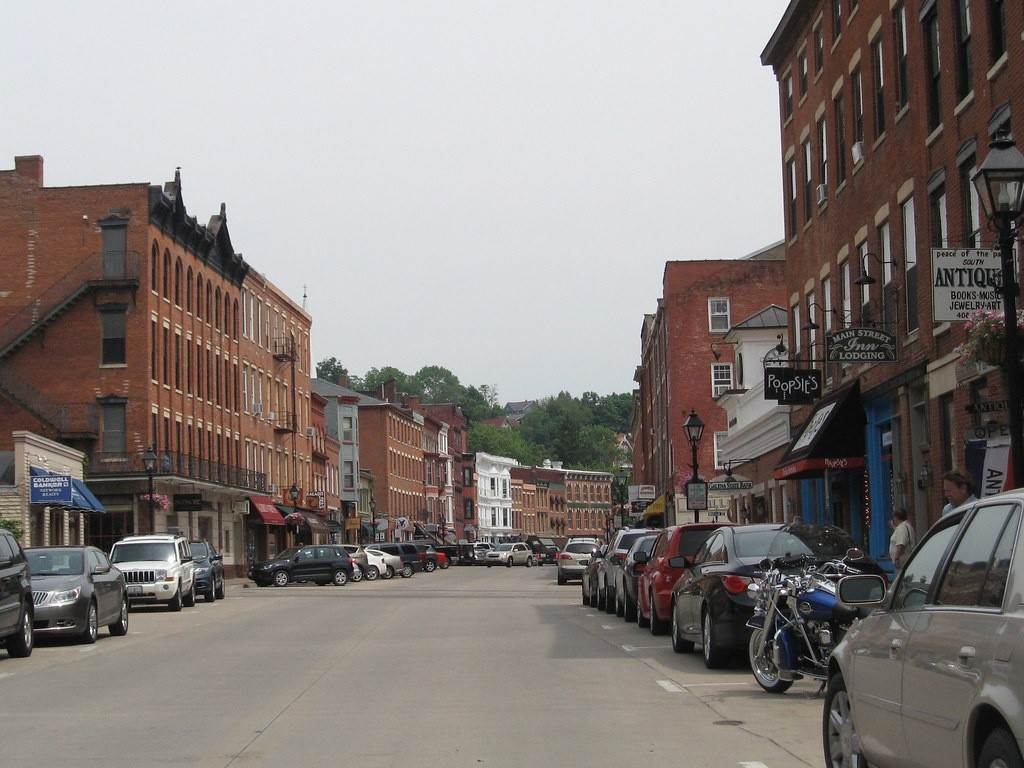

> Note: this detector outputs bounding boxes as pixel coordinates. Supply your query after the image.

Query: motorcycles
[746,547,874,694]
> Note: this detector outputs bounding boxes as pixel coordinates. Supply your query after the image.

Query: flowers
[613,502,632,516]
[951,307,1024,370]
[368,520,380,527]
[284,512,304,525]
[139,494,170,512]
[679,472,705,496]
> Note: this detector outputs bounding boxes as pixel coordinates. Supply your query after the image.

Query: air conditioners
[851,140,864,166]
[306,427,316,437]
[816,184,828,206]
[252,404,262,414]
[267,411,275,421]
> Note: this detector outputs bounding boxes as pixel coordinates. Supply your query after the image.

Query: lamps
[775,333,787,354]
[710,341,739,361]
[854,252,898,286]
[801,302,837,331]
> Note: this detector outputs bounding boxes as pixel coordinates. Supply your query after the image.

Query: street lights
[604,509,611,544]
[441,516,446,546]
[368,496,377,544]
[682,407,706,523]
[969,125,1024,489]
[141,446,158,535]
[615,466,627,528]
[288,482,300,546]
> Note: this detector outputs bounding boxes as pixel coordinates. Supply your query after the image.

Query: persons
[942,469,977,517]
[793,516,802,523]
[888,508,916,577]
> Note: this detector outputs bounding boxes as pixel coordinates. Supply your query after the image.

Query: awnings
[360,522,379,536]
[30,466,106,513]
[244,496,285,525]
[773,382,865,481]
[275,505,331,534]
[642,493,664,515]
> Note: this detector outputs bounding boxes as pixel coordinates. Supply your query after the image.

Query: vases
[976,336,1024,368]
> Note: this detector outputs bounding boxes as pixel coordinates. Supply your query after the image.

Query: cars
[581,521,888,669]
[533,536,604,585]
[297,539,496,583]
[0,529,129,658]
[822,488,1024,768]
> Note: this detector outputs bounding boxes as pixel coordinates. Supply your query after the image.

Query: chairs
[68,554,84,570]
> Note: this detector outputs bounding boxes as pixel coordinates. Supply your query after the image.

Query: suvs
[485,542,533,568]
[108,531,225,613]
[246,544,355,587]
